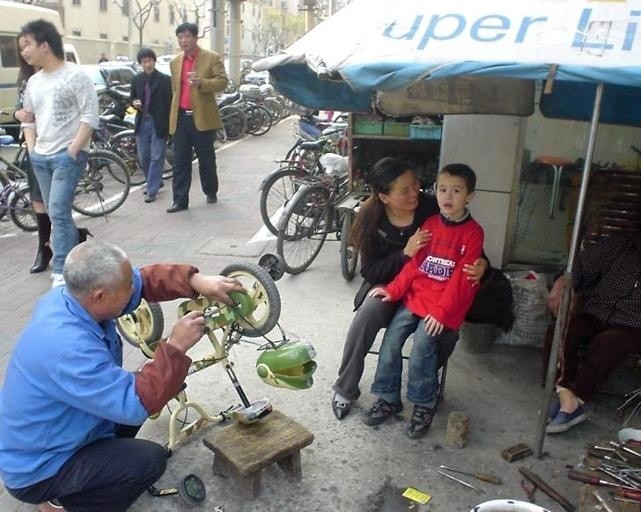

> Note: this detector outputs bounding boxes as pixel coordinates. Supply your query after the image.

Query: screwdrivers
[441,465,501,484]
[588,448,627,465]
[567,471,641,492]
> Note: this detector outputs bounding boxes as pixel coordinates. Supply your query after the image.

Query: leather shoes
[207,194,217,203]
[167,203,187,212]
[333,393,351,419]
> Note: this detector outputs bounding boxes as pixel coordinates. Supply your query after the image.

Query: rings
[417,241,420,245]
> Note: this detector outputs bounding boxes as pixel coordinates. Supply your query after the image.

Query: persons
[362,164,484,438]
[24,20,99,288]
[537,231,641,433]
[130,49,171,202]
[0,241,246,512]
[331,157,490,420]
[13,34,93,272]
[167,23,228,212]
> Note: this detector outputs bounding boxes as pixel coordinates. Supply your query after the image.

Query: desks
[514,429,641,511]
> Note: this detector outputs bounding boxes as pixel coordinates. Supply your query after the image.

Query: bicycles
[259,100,367,286]
[0,83,259,231]
[113,251,321,499]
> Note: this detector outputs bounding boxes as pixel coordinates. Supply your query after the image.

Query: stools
[198,408,318,499]
[365,312,449,405]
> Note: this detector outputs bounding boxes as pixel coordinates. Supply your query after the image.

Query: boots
[30,213,53,273]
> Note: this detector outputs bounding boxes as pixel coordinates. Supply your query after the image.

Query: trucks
[0,2,79,143]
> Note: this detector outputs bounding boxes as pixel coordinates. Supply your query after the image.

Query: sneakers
[409,405,436,438]
[362,398,403,426]
[546,400,587,433]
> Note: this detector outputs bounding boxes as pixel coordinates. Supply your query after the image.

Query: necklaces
[388,212,412,236]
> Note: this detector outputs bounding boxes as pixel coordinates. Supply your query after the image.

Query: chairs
[537,171,641,391]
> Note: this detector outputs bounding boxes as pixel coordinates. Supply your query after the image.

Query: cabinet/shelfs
[347,109,442,199]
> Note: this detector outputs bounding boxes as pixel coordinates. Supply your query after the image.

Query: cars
[82,55,273,93]
